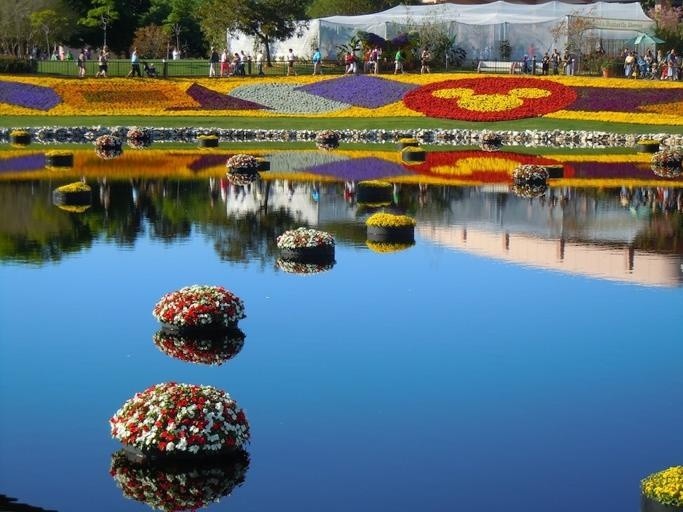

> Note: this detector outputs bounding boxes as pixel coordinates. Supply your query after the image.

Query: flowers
[223,152,262,170]
[277,227,334,249]
[46,148,75,161]
[153,285,247,327]
[110,451,250,512]
[365,213,417,227]
[94,135,122,147]
[124,129,154,140]
[153,330,246,366]
[314,128,339,145]
[10,128,31,138]
[366,240,415,253]
[358,180,392,187]
[358,202,390,208]
[109,382,249,454]
[195,132,220,142]
[276,258,336,273]
[398,133,683,197]
[640,466,683,507]
[54,180,94,196]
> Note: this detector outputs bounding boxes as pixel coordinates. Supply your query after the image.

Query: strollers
[142,64,160,77]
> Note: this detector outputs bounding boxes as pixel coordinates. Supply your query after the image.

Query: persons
[311,48,323,75]
[30,45,110,78]
[393,48,408,75]
[208,47,267,78]
[343,46,384,75]
[521,46,683,81]
[131,51,141,77]
[286,48,297,76]
[126,47,138,77]
[82,177,683,226]
[172,48,180,61]
[419,47,431,74]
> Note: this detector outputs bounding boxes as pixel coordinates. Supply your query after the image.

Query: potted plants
[601,61,619,77]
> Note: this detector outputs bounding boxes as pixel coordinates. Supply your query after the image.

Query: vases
[357,188,393,203]
[161,321,239,337]
[123,444,243,466]
[367,227,414,240]
[280,249,335,264]
[642,496,683,512]
